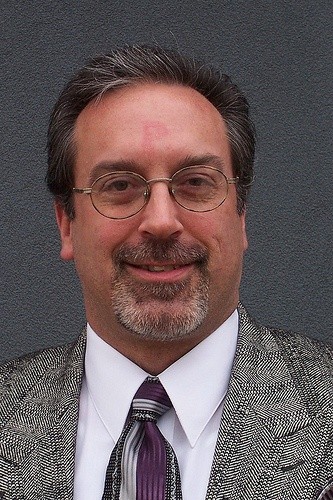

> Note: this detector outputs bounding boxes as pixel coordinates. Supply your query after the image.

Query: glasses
[71,171,241,219]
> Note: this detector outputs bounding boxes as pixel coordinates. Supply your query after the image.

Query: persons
[0,40,333,500]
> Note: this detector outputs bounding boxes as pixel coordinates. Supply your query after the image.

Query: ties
[101,378,183,500]
